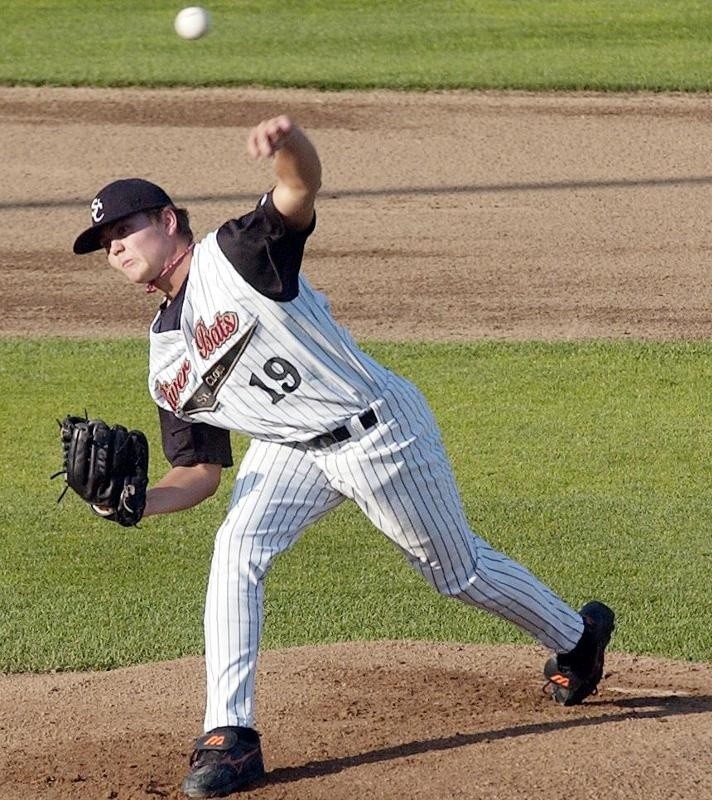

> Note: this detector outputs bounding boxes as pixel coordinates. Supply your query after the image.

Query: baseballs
[173,7,209,40]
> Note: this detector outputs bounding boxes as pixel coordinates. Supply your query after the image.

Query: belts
[305,409,378,451]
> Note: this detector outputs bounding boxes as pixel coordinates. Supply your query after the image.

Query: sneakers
[540,601,616,707]
[180,726,267,796]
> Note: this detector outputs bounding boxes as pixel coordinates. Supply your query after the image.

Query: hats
[72,178,177,255]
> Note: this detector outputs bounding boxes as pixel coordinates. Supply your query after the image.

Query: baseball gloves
[60,414,150,527]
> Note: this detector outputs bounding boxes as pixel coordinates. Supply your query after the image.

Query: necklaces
[144,242,195,293]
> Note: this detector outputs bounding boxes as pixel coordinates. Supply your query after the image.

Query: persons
[50,114,615,798]
